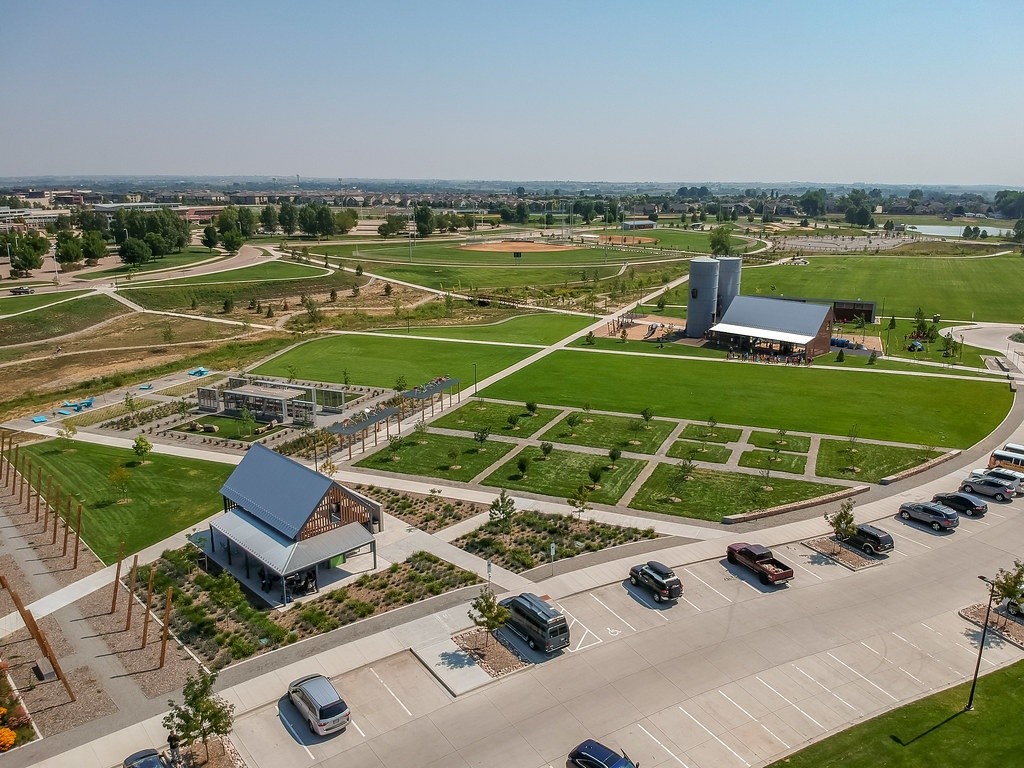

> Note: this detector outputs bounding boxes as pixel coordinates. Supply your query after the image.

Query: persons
[726,343,815,368]
[257,567,317,603]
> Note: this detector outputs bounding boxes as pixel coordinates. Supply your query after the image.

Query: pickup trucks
[727,542,795,587]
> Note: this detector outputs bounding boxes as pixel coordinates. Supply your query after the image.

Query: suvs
[496,592,569,655]
[835,525,896,557]
[899,442,1024,532]
[630,561,683,603]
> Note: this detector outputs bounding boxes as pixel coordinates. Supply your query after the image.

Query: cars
[121,749,166,768]
[567,739,640,768]
[288,671,350,737]
[1002,584,1024,615]
